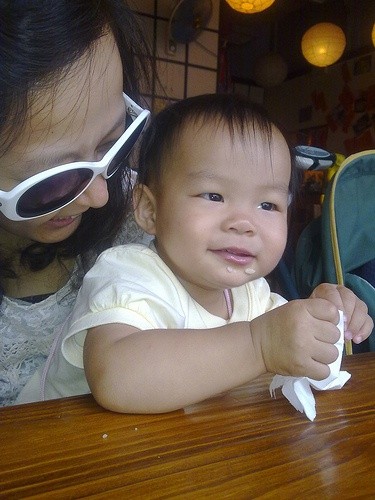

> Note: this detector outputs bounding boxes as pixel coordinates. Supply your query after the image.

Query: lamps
[301,23,347,67]
[226,0,276,14]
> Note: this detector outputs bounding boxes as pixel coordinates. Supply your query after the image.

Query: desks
[0,351,375,500]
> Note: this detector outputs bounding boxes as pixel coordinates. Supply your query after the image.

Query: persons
[13,94,375,407]
[1,0,152,409]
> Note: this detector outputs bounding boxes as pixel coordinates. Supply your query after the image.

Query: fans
[165,0,214,56]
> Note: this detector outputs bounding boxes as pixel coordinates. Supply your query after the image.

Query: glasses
[0,89,151,222]
